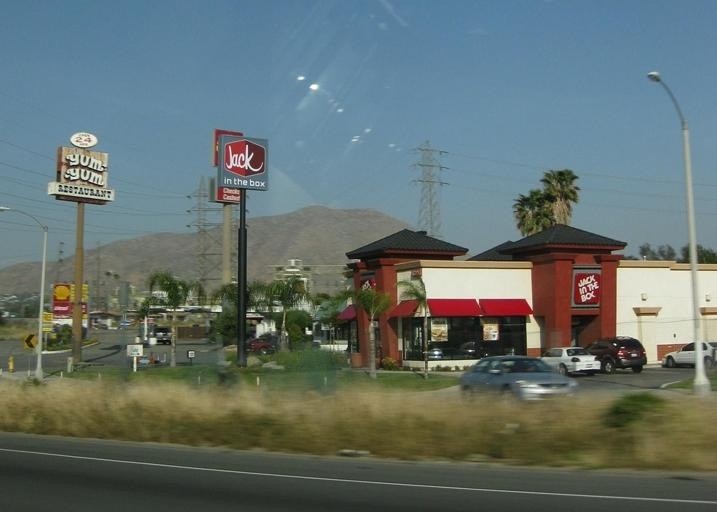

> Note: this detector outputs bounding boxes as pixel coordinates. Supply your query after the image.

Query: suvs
[586,336,647,373]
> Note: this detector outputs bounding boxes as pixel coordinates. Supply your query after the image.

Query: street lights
[646,71,712,395]
[0,206,48,384]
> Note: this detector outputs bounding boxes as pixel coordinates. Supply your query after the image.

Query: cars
[461,342,482,360]
[662,339,716,367]
[245,332,280,356]
[460,355,580,406]
[536,346,602,375]
[502,344,523,356]
[425,348,443,359]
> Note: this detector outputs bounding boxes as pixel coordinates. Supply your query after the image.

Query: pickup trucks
[148,325,171,344]
